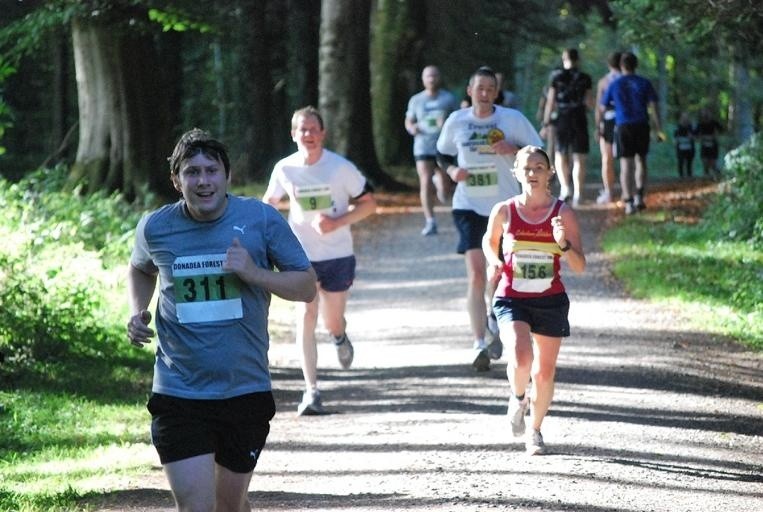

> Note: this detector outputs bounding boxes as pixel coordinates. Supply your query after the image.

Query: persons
[433,66,544,371]
[483,146,586,455]
[459,47,725,215]
[403,64,461,236]
[126,129,318,511]
[259,106,379,416]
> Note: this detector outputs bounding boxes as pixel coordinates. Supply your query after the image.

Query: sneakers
[297,391,322,415]
[557,186,647,216]
[422,224,437,237]
[508,397,528,436]
[332,333,353,369]
[473,348,490,371]
[486,316,503,358]
[526,428,546,455]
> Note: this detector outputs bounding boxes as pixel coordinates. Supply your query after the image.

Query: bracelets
[559,238,571,251]
